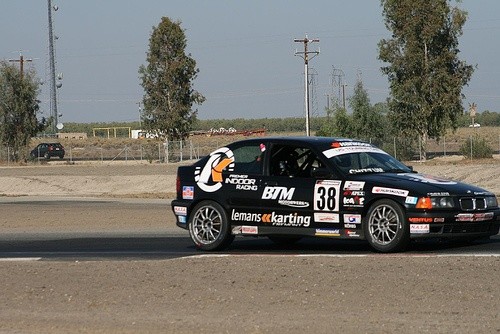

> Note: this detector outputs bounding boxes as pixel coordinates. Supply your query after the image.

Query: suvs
[29,143,65,160]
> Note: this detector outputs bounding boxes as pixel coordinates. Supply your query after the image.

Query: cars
[172,134,500,254]
